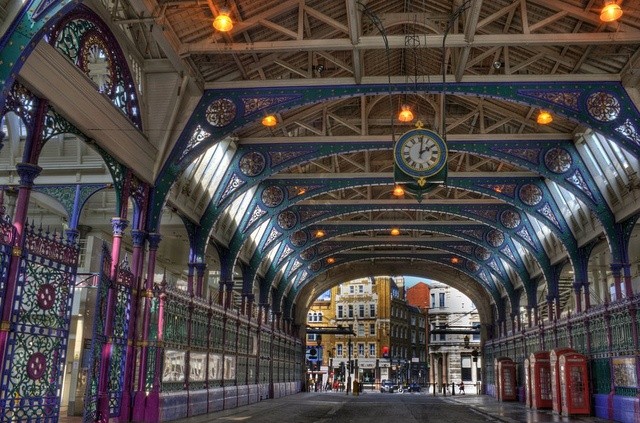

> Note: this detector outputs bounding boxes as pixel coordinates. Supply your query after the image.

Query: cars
[380,380,396,393]
[402,383,421,392]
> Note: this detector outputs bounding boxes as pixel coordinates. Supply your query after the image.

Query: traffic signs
[471,350,478,362]
[309,349,317,359]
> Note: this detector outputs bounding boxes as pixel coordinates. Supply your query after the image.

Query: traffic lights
[350,360,354,374]
[347,360,349,370]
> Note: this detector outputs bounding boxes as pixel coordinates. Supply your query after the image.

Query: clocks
[393,120,449,186]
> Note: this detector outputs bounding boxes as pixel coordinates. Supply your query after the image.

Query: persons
[458,380,465,394]
